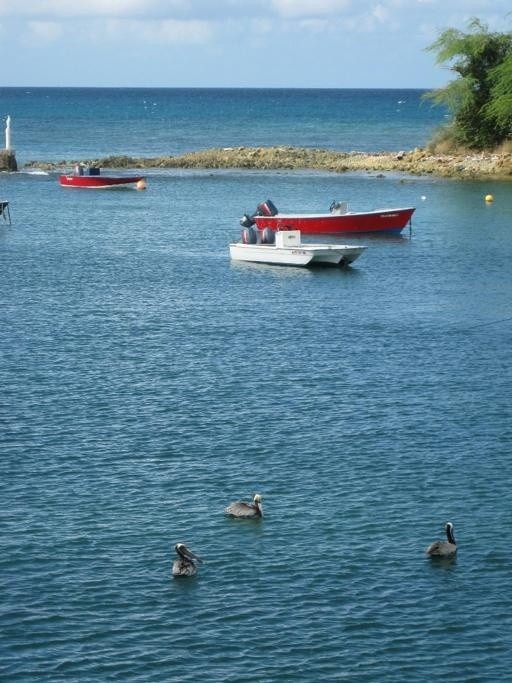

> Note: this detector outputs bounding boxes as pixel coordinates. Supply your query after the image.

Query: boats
[228,208,416,267]
[59,176,144,189]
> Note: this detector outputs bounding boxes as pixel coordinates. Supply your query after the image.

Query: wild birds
[426,521,458,556]
[223,493,264,519]
[171,543,202,576]
